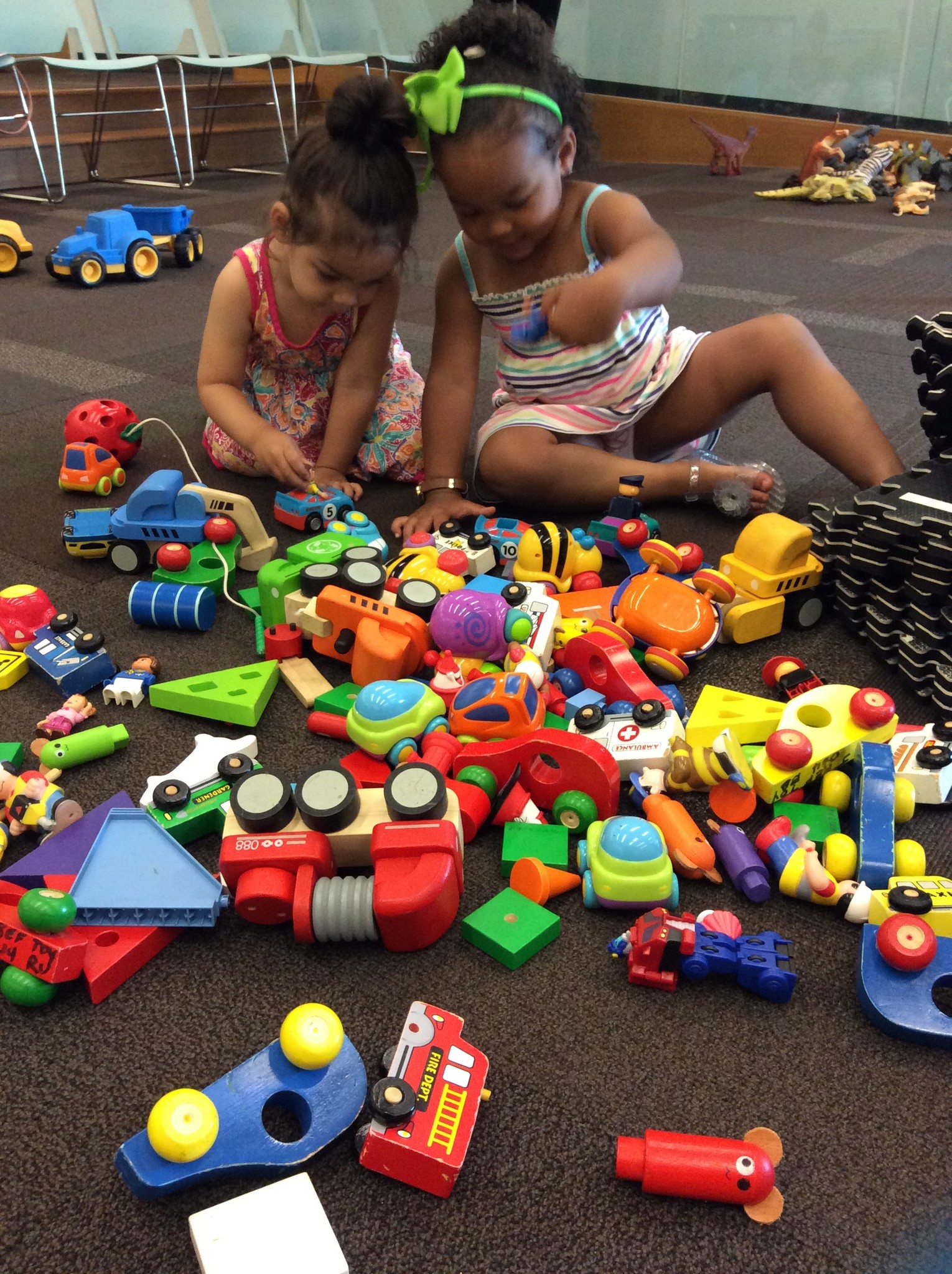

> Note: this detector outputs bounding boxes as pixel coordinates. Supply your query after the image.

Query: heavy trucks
[44,204,204,288]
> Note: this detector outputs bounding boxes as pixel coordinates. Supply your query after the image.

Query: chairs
[0,0,438,203]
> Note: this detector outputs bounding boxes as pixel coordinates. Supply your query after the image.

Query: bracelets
[415,478,470,497]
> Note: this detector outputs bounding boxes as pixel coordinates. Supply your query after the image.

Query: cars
[273,485,358,534]
[59,442,127,496]
[1,512,952,1197]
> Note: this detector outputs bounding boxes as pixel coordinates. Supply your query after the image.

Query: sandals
[682,448,786,515]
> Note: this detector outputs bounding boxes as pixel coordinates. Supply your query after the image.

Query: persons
[197,76,425,500]
[390,11,909,542]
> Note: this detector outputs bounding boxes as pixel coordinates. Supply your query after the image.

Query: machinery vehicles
[62,469,279,577]
